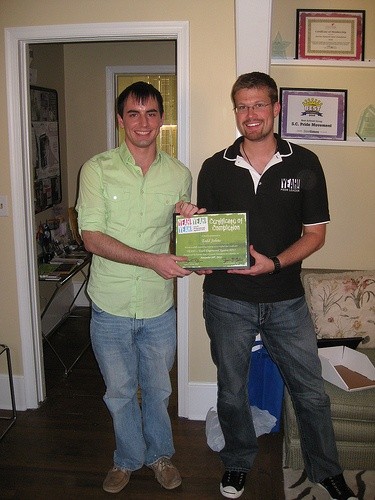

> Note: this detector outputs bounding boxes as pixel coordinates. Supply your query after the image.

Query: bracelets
[268,256,281,275]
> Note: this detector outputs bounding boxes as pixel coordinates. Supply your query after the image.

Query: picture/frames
[294,8,366,61]
[278,87,348,142]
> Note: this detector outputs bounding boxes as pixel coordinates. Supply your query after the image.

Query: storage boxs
[176,212,250,270]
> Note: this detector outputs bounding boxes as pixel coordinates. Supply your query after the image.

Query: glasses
[234,102,272,113]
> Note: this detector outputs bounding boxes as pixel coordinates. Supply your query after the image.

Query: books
[39,251,89,281]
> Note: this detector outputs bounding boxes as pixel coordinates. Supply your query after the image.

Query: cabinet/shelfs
[233,0,375,149]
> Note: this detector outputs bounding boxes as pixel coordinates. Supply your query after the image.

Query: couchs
[286,268,375,469]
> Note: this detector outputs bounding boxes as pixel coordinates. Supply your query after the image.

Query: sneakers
[219,470,246,498]
[149,457,182,489]
[103,466,132,492]
[317,473,358,500]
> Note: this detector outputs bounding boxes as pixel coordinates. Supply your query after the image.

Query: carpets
[282,442,375,500]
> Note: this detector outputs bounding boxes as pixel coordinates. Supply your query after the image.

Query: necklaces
[241,143,262,175]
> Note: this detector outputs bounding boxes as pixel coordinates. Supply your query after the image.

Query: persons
[75,81,207,493]
[194,72,358,500]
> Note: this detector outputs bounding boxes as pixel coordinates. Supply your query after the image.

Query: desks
[36,242,91,373]
